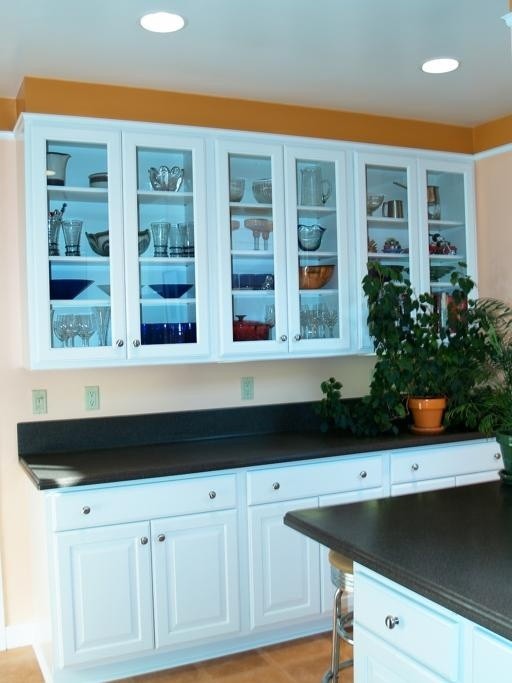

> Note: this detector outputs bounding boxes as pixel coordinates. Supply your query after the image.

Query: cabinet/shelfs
[216,128,352,369]
[390,435,506,502]
[354,142,476,356]
[246,451,388,651]
[14,114,215,371]
[354,562,512,682]
[31,460,244,682]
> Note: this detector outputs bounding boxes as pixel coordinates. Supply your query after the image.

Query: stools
[322,551,353,683]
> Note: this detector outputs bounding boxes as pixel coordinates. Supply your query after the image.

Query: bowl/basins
[404,266,456,282]
[252,179,272,204]
[230,177,245,202]
[86,231,150,257]
[51,279,94,300]
[149,284,194,298]
[299,265,335,289]
[89,173,108,188]
[367,195,385,216]
[98,284,144,297]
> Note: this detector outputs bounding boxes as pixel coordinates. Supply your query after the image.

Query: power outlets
[241,377,255,399]
[86,386,100,411]
[35,390,47,415]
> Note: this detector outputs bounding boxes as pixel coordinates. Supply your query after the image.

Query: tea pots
[47,152,72,186]
[300,166,333,206]
[382,200,404,218]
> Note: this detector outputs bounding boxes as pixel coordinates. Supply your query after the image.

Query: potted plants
[320,258,499,434]
[443,296,512,484]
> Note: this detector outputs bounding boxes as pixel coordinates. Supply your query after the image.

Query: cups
[48,216,61,255]
[54,321,69,348]
[301,303,338,338]
[63,316,81,348]
[168,223,185,257]
[58,315,73,348]
[61,220,83,256]
[151,222,170,257]
[75,315,96,347]
[186,225,194,257]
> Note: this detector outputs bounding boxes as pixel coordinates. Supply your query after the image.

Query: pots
[233,314,270,341]
[393,181,440,206]
